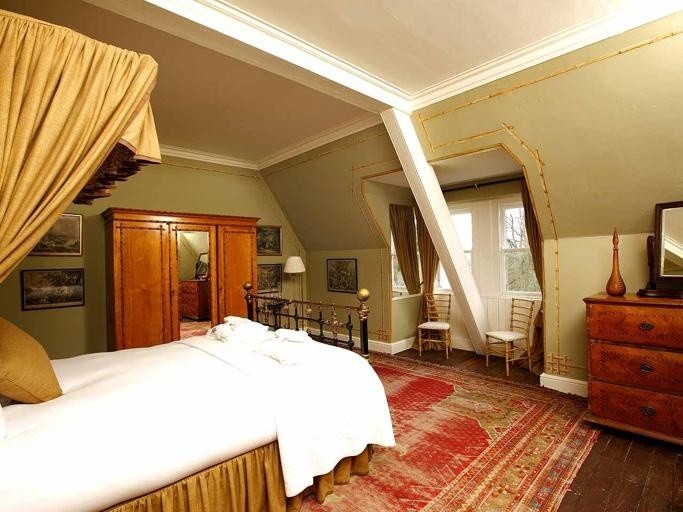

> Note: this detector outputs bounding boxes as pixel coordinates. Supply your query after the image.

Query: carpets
[286,344,601,512]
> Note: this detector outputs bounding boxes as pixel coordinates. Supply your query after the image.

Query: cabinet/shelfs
[180,279,210,321]
[101,205,261,352]
[581,290,683,446]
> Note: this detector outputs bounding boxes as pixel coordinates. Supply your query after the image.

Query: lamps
[282,256,307,280]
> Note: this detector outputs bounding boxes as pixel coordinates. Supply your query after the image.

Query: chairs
[485,296,535,377]
[417,292,452,361]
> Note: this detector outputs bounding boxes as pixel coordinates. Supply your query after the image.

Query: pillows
[2,316,63,403]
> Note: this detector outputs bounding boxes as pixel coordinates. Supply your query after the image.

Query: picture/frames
[19,267,86,310]
[256,224,283,256]
[326,257,359,294]
[256,264,282,296]
[26,212,84,259]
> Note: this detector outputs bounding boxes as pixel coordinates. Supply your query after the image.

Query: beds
[1,281,397,512]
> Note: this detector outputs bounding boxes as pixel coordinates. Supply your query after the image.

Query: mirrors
[196,253,210,277]
[170,224,219,341]
[638,199,683,300]
